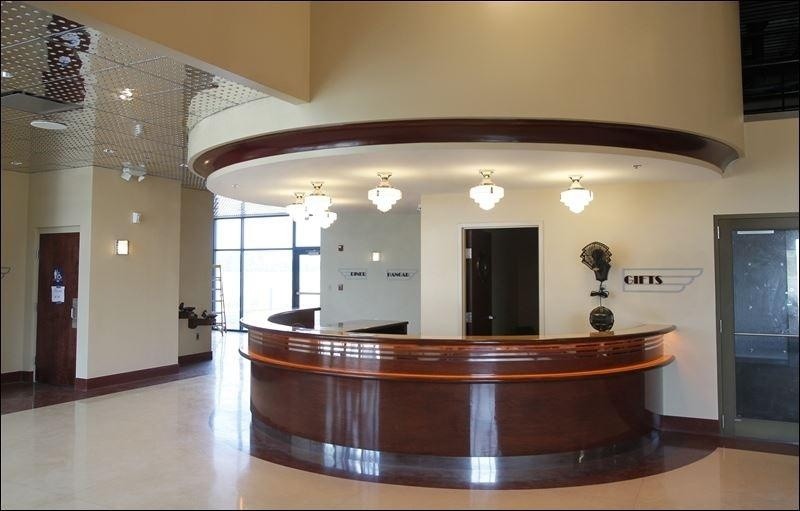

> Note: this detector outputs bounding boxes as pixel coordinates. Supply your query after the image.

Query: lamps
[283,180,339,231]
[366,169,593,213]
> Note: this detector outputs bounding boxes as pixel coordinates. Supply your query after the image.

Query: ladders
[212,265,227,336]
